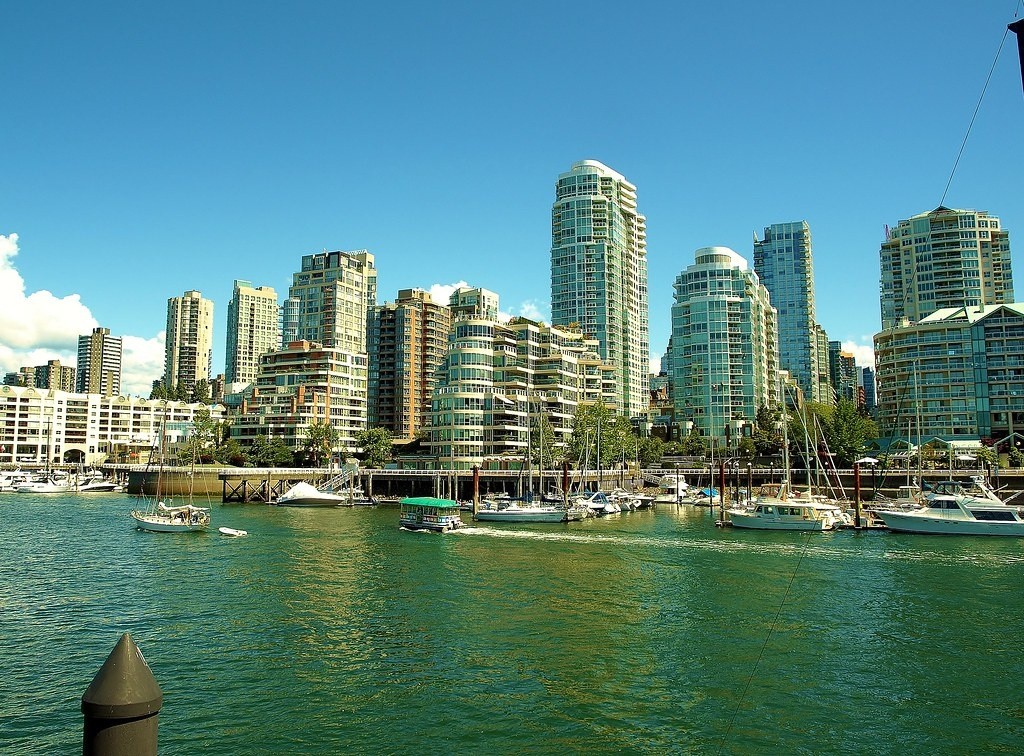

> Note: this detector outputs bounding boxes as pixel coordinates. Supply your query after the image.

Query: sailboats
[128,387,213,533]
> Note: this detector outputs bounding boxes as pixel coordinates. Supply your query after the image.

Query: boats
[276,482,344,508]
[218,526,248,537]
[0,414,120,494]
[469,374,1024,542]
[398,496,462,533]
